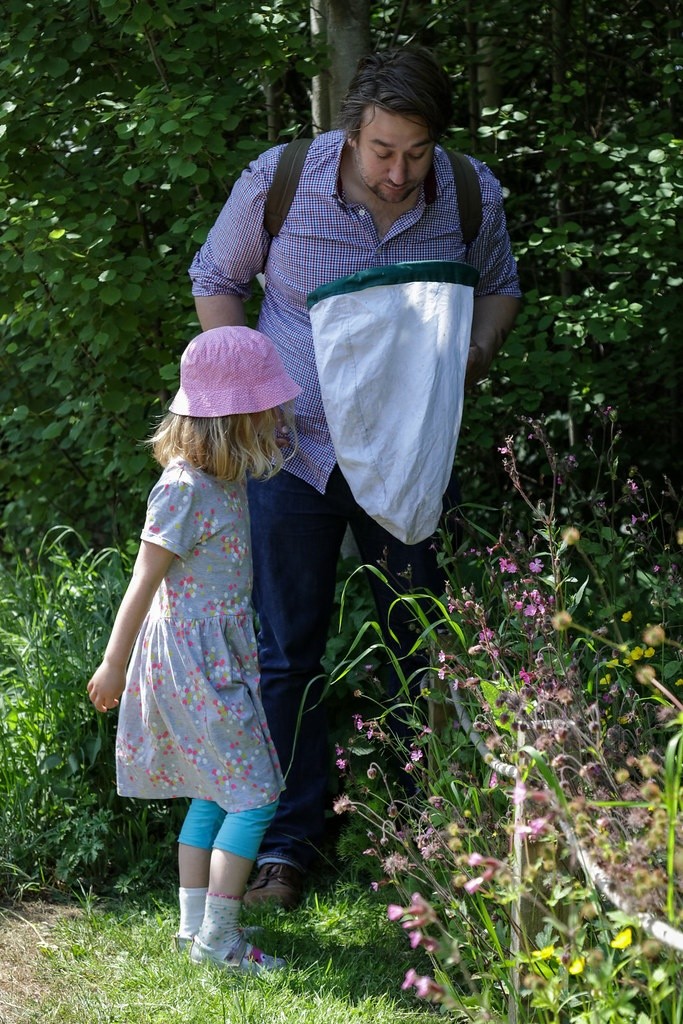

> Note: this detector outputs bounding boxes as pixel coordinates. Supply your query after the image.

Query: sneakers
[244,864,302,911]
[190,935,286,975]
[174,926,262,954]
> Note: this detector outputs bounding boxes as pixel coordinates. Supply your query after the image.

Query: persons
[88,327,304,975]
[185,37,524,915]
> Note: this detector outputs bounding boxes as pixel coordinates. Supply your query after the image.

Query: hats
[170,325,304,416]
